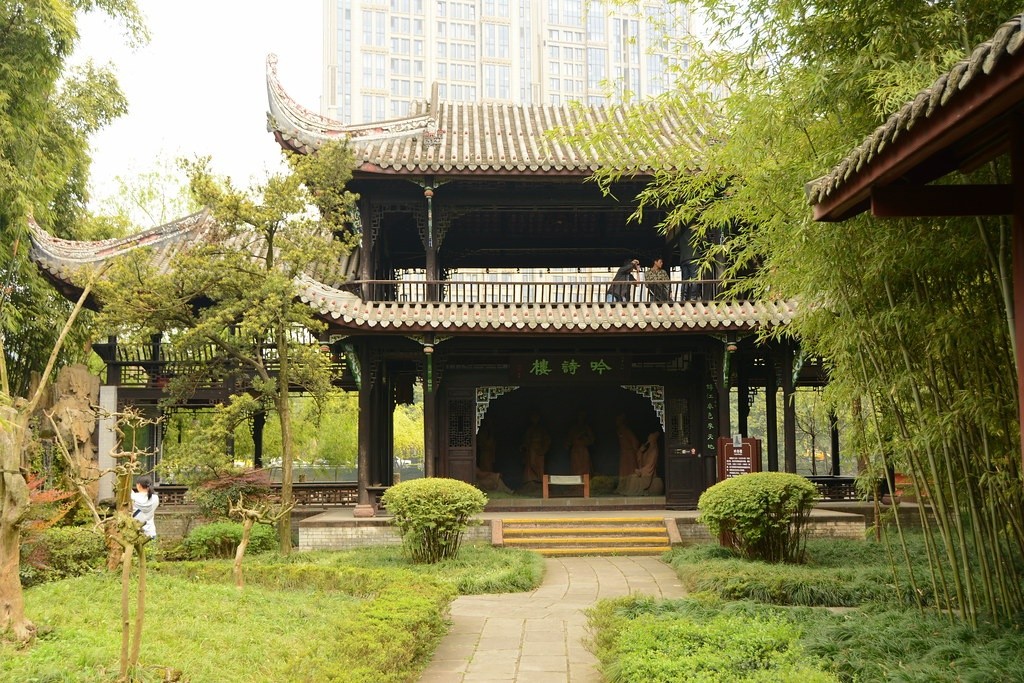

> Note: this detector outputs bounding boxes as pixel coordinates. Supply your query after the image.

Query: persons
[615,414,639,476]
[477,415,496,472]
[518,412,551,497]
[679,220,704,301]
[565,418,594,478]
[615,431,662,498]
[645,257,673,301]
[131,476,159,541]
[606,258,640,302]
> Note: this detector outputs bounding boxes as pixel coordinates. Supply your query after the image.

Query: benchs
[542,473,591,497]
